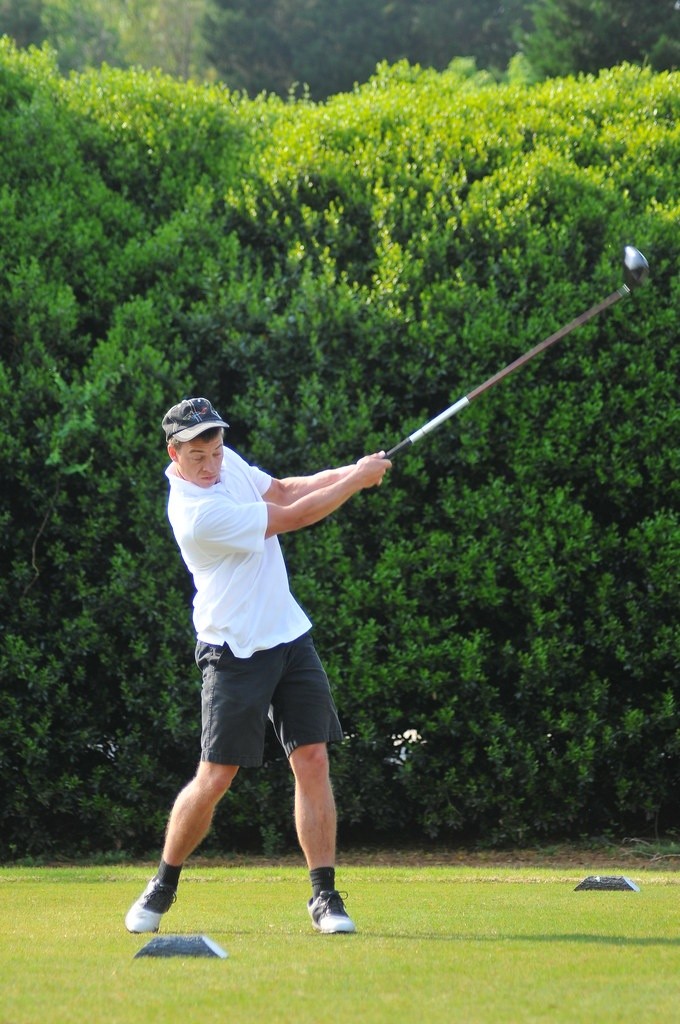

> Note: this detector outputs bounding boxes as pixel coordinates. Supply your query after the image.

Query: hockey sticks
[378,244,650,459]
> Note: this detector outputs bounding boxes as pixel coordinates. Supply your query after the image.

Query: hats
[161,398,229,442]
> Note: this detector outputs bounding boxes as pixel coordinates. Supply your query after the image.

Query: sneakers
[124,876,177,934]
[307,889,355,933]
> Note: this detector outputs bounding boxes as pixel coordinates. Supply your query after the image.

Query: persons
[123,396,392,935]
